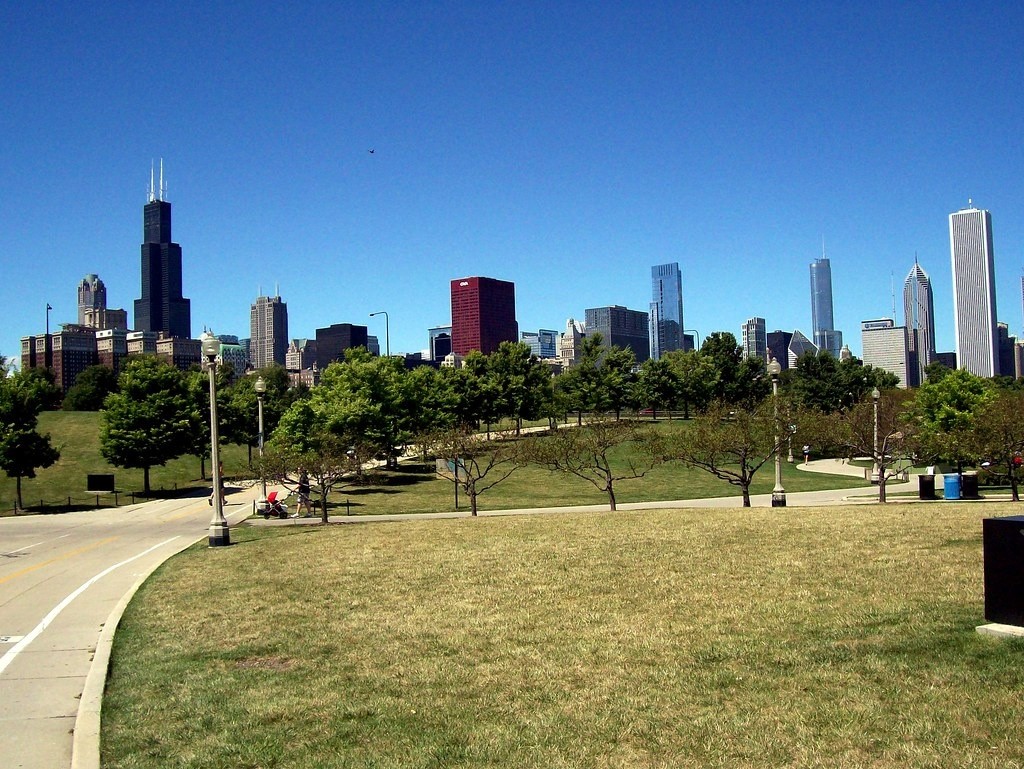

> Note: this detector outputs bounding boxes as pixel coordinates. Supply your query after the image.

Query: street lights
[769,357,786,506]
[46,302,52,382]
[201,328,231,546]
[871,387,880,484]
[785,402,793,464]
[255,376,271,516]
[370,311,390,357]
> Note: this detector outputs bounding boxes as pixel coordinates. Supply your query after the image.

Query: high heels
[305,513,312,518]
[291,514,300,519]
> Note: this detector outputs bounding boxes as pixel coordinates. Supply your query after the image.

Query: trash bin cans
[943,473,961,500]
[918,473,937,500]
[963,474,980,500]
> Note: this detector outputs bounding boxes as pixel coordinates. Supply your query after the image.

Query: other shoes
[222,501,228,505]
[209,499,213,506]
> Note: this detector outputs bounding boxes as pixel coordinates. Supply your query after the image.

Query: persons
[802,442,810,465]
[925,461,936,475]
[1012,448,1023,471]
[208,461,228,506]
[290,466,312,518]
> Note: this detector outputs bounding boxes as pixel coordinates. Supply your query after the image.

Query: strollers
[262,491,291,519]
